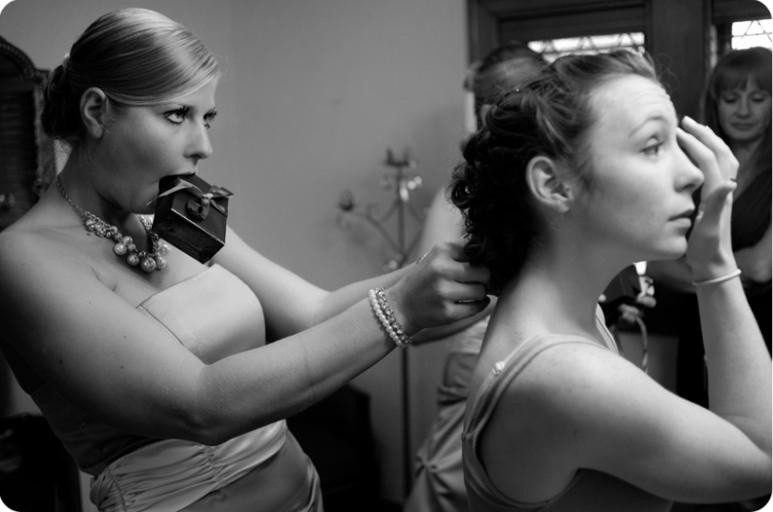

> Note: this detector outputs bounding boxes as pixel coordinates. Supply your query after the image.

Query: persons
[443,49,772,512]
[647,48,772,406]
[404,42,657,511]
[0,8,490,512]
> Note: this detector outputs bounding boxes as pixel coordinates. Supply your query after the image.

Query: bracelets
[377,288,413,344]
[367,289,404,348]
[690,269,742,286]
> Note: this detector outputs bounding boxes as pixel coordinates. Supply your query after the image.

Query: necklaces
[55,175,170,273]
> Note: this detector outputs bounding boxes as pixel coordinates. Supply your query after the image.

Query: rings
[731,177,736,182]
